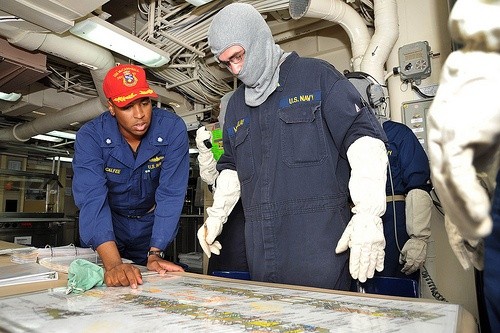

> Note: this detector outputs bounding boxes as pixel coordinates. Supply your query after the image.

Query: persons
[196,90,250,283]
[337,70,433,299]
[71,64,190,290]
[194,3,392,293]
[424,0,500,333]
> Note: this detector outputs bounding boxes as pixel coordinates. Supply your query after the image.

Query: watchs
[146,250,165,259]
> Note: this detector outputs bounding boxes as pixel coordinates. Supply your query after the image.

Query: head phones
[345,72,385,108]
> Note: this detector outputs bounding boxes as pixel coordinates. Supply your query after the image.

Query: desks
[0,240,478,333]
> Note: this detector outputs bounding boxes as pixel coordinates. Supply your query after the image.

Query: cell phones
[198,124,212,149]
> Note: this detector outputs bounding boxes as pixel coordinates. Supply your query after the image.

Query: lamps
[69,16,171,68]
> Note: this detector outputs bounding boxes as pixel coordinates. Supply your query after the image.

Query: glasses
[217,48,246,69]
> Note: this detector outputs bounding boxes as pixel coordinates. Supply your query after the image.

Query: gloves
[197,169,241,258]
[196,126,220,186]
[427,0,500,248]
[335,136,388,283]
[443,213,485,271]
[399,189,433,275]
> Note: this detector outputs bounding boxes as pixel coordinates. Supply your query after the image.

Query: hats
[102,64,158,107]
